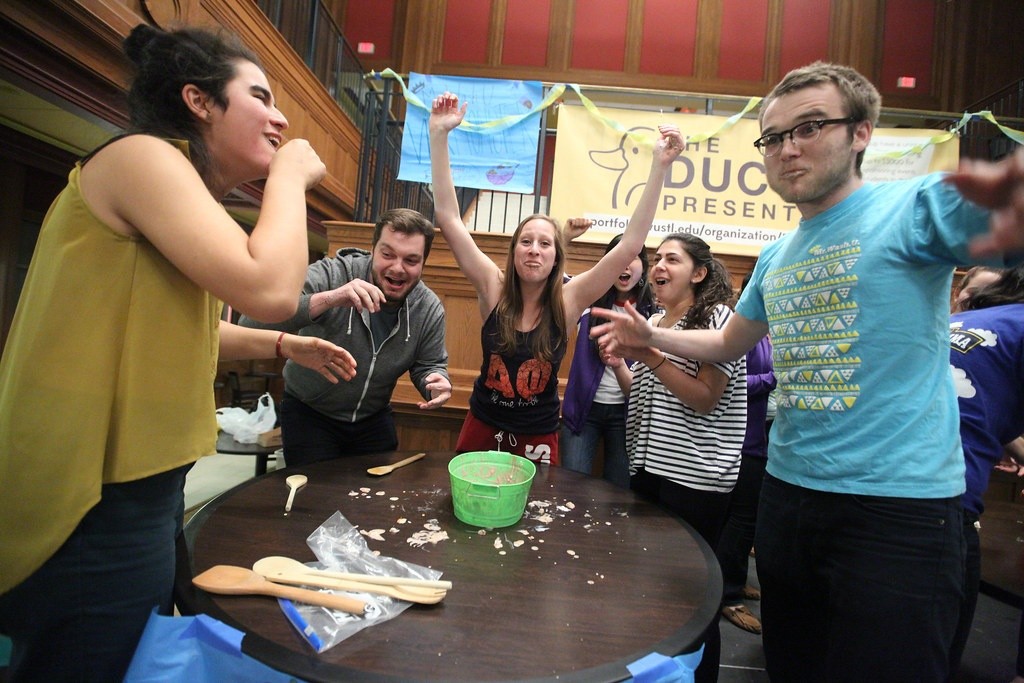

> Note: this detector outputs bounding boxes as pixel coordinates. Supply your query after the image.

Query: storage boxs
[258,426,282,448]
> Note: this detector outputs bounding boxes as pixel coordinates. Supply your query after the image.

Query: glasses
[753,117,856,157]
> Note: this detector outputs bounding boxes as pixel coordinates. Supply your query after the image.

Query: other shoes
[721,604,763,635]
[743,583,762,602]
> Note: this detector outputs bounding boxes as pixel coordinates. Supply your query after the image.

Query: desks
[174,451,724,683]
[217,442,281,477]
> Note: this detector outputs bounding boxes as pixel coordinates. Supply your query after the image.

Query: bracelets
[276,331,287,359]
[650,355,666,371]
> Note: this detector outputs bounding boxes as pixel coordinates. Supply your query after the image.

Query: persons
[1,23,329,683]
[236,208,453,467]
[429,90,685,473]
[559,59,1024,683]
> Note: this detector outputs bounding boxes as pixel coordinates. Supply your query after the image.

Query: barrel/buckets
[448,451,535,528]
